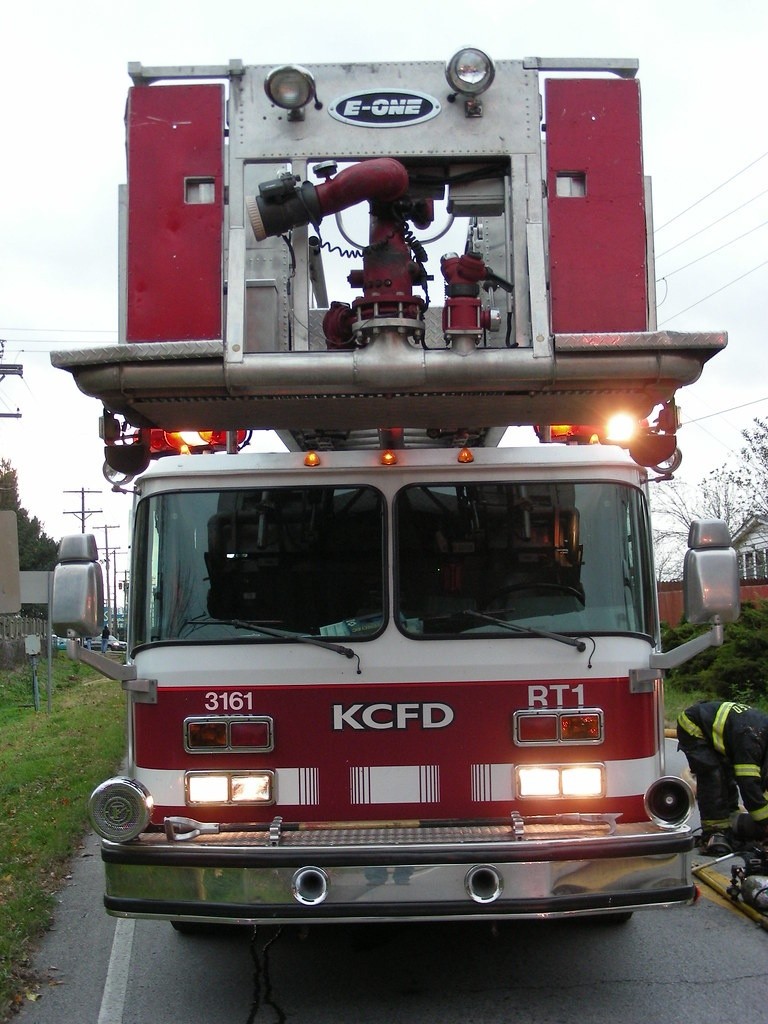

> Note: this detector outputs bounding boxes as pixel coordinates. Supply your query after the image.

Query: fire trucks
[51,47,742,937]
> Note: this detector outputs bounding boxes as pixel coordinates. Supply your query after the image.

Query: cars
[83,634,128,651]
[58,638,71,650]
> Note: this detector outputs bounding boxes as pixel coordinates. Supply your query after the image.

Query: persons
[676,700,768,858]
[101,626,110,653]
[86,638,92,650]
[485,523,569,612]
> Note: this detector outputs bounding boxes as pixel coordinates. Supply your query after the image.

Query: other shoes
[700,831,713,843]
